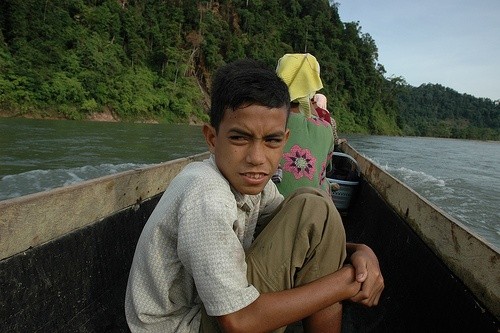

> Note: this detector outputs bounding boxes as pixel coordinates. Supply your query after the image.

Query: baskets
[325,152,361,210]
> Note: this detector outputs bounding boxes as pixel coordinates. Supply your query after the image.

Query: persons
[125,60,384,333]
[272,53,346,200]
